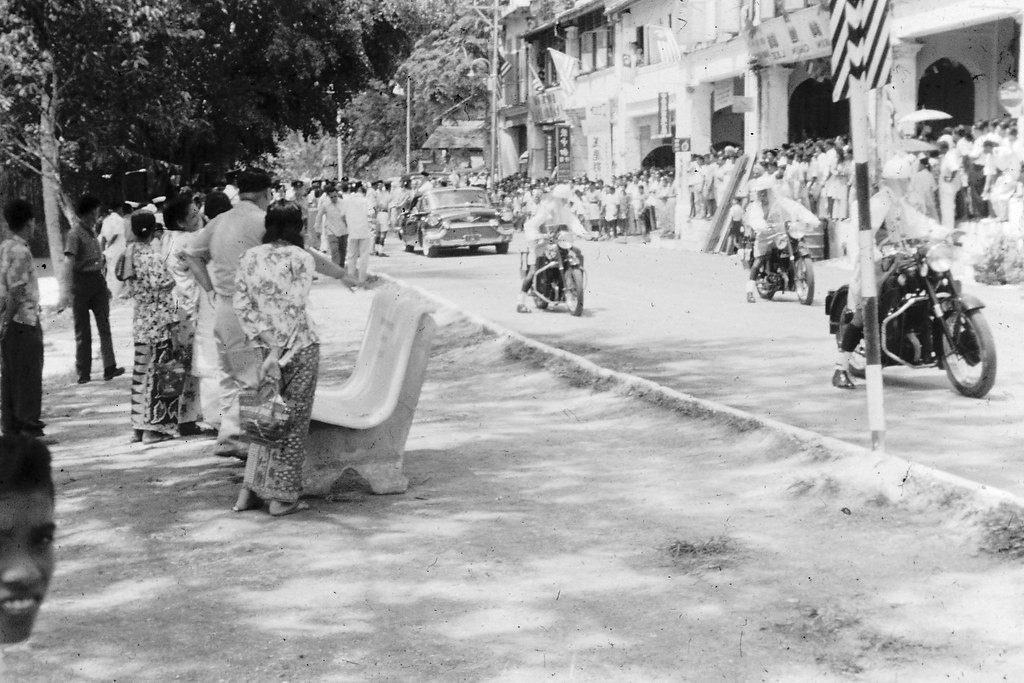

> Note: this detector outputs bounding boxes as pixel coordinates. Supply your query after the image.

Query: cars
[398,185,515,258]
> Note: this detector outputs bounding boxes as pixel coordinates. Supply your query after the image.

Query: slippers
[271,500,309,516]
[233,498,264,511]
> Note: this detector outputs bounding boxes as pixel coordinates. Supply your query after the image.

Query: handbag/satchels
[238,374,290,449]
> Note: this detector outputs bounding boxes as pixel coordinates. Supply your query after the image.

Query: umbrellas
[897,105,954,124]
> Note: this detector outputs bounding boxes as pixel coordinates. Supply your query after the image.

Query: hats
[552,185,570,199]
[237,167,272,192]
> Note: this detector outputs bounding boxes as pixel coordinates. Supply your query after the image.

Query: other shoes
[143,434,173,442]
[747,292,755,302]
[104,367,124,381]
[516,304,532,313]
[78,376,90,383]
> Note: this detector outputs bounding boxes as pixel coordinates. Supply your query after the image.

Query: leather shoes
[832,370,854,389]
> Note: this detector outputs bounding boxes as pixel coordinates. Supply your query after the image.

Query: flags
[532,70,545,93]
[497,52,512,99]
[651,25,682,66]
[550,50,574,97]
[830,0,891,103]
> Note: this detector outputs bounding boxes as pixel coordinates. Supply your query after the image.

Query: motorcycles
[737,218,815,305]
[520,225,588,315]
[824,237,997,398]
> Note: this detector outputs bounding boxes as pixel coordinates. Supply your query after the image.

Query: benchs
[299,292,439,497]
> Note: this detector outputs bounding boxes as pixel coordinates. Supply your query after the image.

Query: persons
[159,193,232,437]
[233,198,320,517]
[93,119,1024,312]
[184,168,361,456]
[116,213,181,442]
[57,194,125,384]
[0,435,56,643]
[833,157,968,389]
[0,199,45,436]
